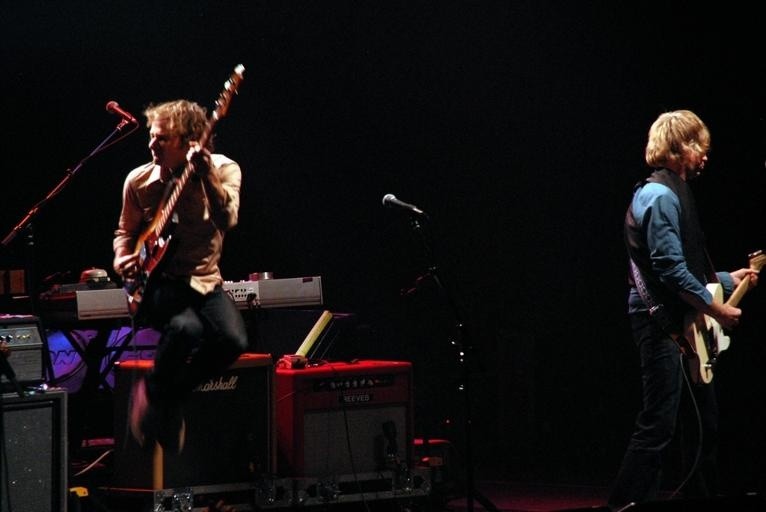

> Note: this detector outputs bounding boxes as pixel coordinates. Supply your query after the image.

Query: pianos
[13,276,324,324]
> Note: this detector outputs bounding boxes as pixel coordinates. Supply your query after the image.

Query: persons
[595,108,761,507]
[108,96,250,442]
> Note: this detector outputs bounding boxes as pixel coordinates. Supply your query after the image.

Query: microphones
[380,194,423,218]
[105,100,137,123]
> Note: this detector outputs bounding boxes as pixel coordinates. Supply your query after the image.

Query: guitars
[123,65,244,319]
[686,250,764,385]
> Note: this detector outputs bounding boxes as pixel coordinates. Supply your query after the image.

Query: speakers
[274,356,416,486]
[112,353,273,497]
[0,387,70,512]
